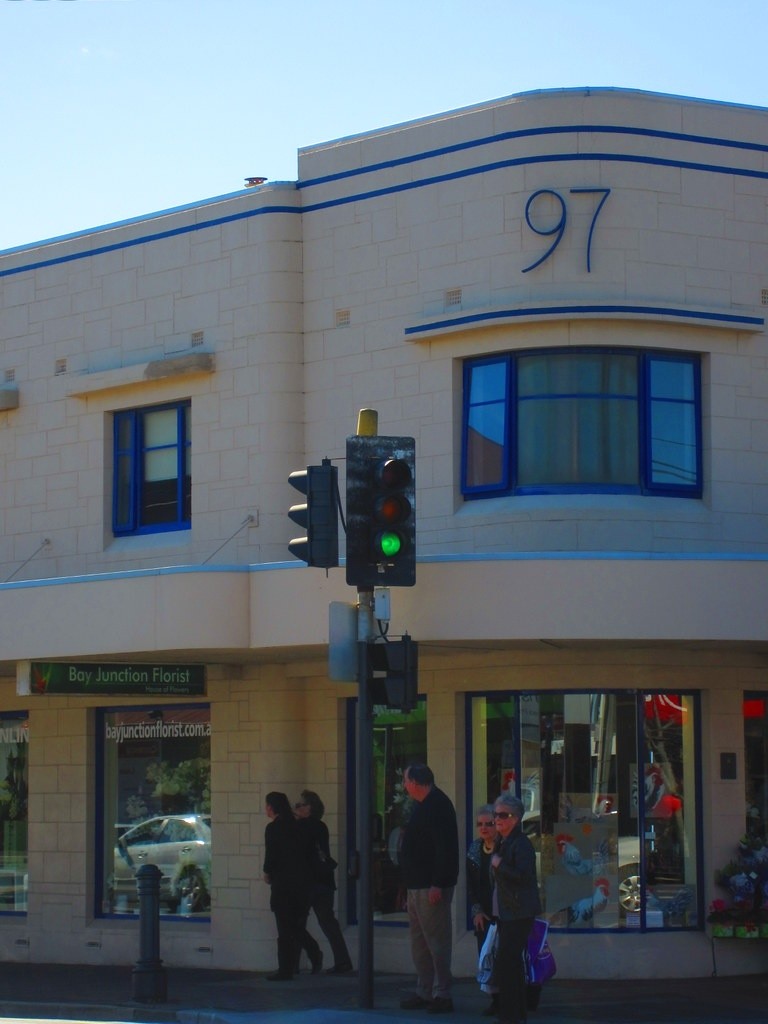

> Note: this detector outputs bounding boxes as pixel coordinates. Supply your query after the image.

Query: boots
[484,993,505,1015]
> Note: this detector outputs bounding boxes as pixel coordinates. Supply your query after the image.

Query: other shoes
[494,1016,527,1024]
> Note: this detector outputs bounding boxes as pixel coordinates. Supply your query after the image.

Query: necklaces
[481,842,494,855]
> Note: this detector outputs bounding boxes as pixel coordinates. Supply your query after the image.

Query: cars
[110,811,214,910]
[522,811,644,922]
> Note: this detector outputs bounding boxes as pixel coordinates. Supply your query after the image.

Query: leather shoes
[326,963,353,975]
[400,994,454,1013]
[265,970,290,981]
[310,952,323,975]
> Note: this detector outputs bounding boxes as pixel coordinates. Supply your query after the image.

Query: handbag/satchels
[477,916,510,995]
[522,919,557,985]
[297,820,338,884]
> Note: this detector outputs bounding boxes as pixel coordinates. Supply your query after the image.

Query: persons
[396,763,459,1012]
[263,790,352,980]
[467,793,543,1024]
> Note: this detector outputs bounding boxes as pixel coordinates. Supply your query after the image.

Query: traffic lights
[365,638,418,715]
[340,433,418,590]
[288,465,339,570]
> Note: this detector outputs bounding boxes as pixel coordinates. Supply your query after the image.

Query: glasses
[476,822,493,826]
[493,812,520,818]
[295,803,311,808]
